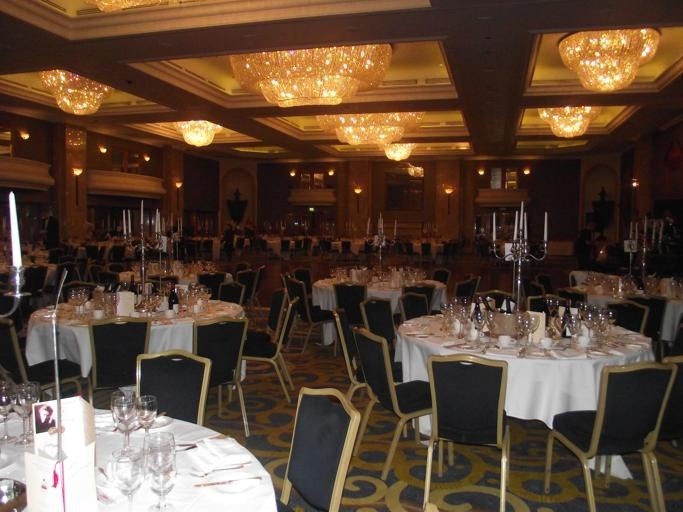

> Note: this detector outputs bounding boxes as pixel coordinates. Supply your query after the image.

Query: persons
[113,223,123,238]
[201,220,255,262]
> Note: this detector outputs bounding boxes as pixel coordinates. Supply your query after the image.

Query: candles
[492,202,548,241]
[630,215,665,244]
[6,192,23,269]
[122,199,183,241]
[365,212,397,239]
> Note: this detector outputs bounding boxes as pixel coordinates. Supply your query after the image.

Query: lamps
[37,71,115,116]
[172,44,423,163]
[535,30,660,139]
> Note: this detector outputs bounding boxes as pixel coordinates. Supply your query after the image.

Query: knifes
[177,433,263,489]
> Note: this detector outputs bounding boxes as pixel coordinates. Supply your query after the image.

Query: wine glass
[586,269,678,301]
[66,282,213,324]
[0,378,39,448]
[108,390,157,445]
[334,265,426,290]
[111,433,177,512]
[439,297,616,356]
[133,260,214,273]
